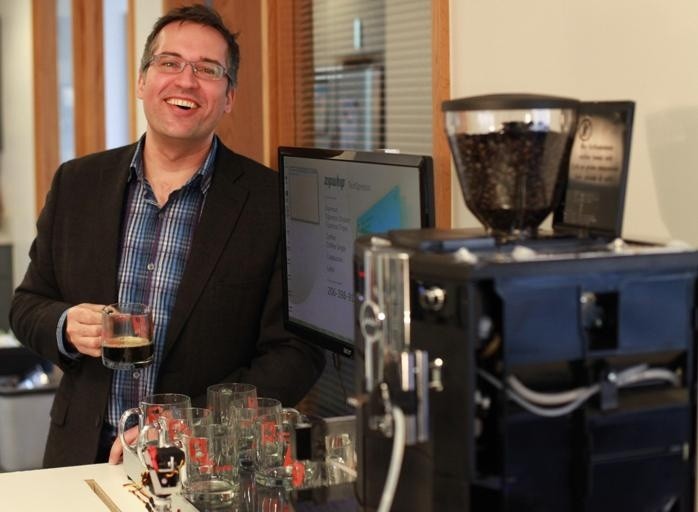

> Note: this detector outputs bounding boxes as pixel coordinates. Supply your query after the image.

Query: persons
[9,6,327,468]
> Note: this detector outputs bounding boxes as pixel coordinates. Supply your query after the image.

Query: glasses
[143,54,236,85]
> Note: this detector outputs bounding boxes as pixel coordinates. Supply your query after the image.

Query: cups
[99,301,156,372]
[118,381,355,512]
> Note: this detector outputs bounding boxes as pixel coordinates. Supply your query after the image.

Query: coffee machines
[348,90,698,512]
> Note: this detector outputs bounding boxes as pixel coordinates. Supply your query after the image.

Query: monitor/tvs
[277,144,436,365]
